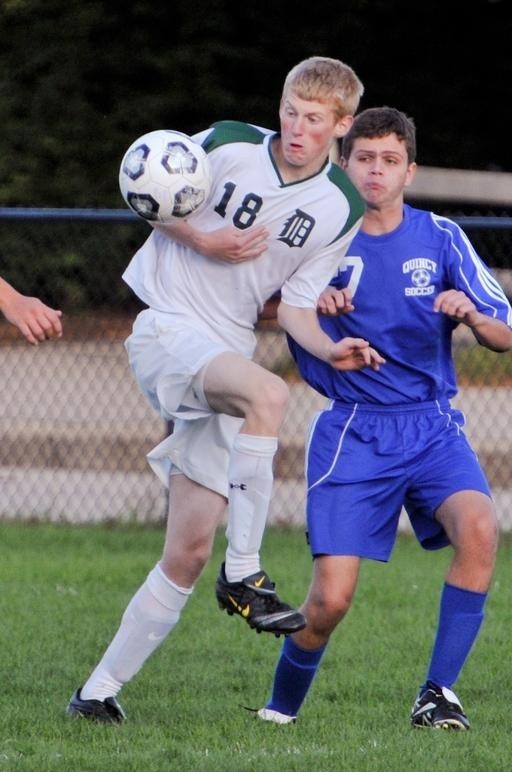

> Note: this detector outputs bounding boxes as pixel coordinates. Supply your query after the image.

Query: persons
[0,278,66,349]
[250,106,510,733]
[63,53,389,730]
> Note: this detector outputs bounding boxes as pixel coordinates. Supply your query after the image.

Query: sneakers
[65,688,128,728]
[410,684,472,731]
[215,562,306,638]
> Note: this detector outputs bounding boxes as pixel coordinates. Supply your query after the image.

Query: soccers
[119,129,212,223]
